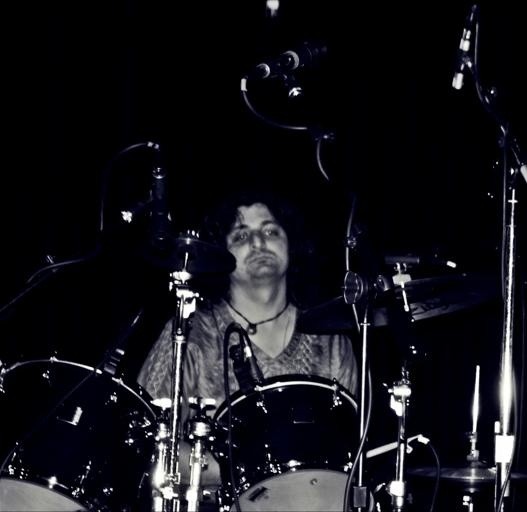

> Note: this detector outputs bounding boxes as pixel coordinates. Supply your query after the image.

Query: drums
[0,349,170,512]
[209,373,360,511]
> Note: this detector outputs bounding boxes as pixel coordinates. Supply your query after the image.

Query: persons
[133,180,411,512]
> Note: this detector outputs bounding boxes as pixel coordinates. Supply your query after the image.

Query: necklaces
[225,299,293,334]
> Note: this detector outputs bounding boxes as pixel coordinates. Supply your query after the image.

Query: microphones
[266,0,304,99]
[247,41,329,77]
[451,8,475,91]
[150,165,172,240]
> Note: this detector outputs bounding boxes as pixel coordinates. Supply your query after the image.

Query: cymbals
[154,236,227,254]
[405,463,527,483]
[310,276,479,326]
